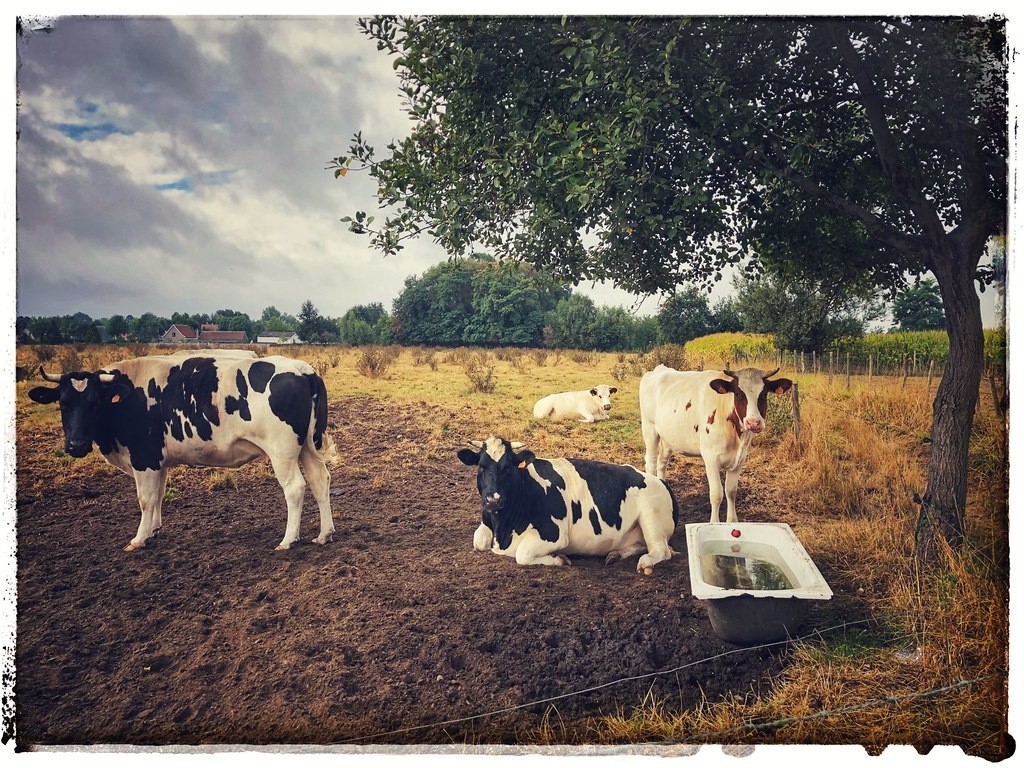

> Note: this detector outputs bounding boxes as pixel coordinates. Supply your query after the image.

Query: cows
[28,349,337,551]
[533,385,617,423]
[639,363,792,522]
[457,436,680,575]
